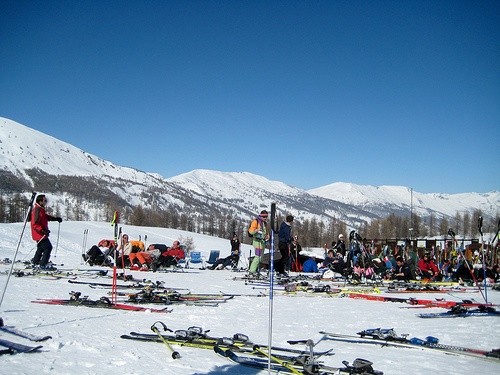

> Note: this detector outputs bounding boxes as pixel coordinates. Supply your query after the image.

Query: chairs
[141,243,167,272]
[164,245,190,272]
[226,250,242,270]
[204,249,221,268]
[84,241,118,265]
[187,251,203,269]
[107,241,145,267]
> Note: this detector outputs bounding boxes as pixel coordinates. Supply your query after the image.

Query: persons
[390,257,413,280]
[207,249,239,270]
[230,233,240,267]
[447,256,460,273]
[491,265,500,283]
[321,249,348,279]
[302,256,319,273]
[278,215,297,278]
[453,260,473,286]
[30,195,62,270]
[247,209,271,276]
[495,248,500,265]
[478,262,496,284]
[391,254,402,269]
[128,244,162,271]
[82,240,110,265]
[440,259,453,282]
[290,238,302,257]
[336,233,346,260]
[417,253,439,282]
[105,234,133,267]
[373,237,382,257]
[152,241,185,272]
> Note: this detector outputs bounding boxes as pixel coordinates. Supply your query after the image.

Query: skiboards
[68,279,188,290]
[18,268,70,276]
[319,331,500,360]
[234,275,341,293]
[385,287,484,292]
[0,325,52,353]
[416,311,500,318]
[213,345,384,375]
[31,299,173,312]
[121,331,333,364]
[107,290,234,307]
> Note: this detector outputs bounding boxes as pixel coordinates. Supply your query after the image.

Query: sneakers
[88,260,93,266]
[42,265,58,271]
[129,267,139,270]
[139,267,148,271]
[82,254,87,262]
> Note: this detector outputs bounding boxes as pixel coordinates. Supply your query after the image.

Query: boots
[206,264,217,270]
[217,265,225,270]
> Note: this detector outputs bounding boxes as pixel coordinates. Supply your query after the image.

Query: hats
[259,210,268,217]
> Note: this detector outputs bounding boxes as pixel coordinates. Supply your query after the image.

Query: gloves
[41,229,50,237]
[55,217,62,223]
